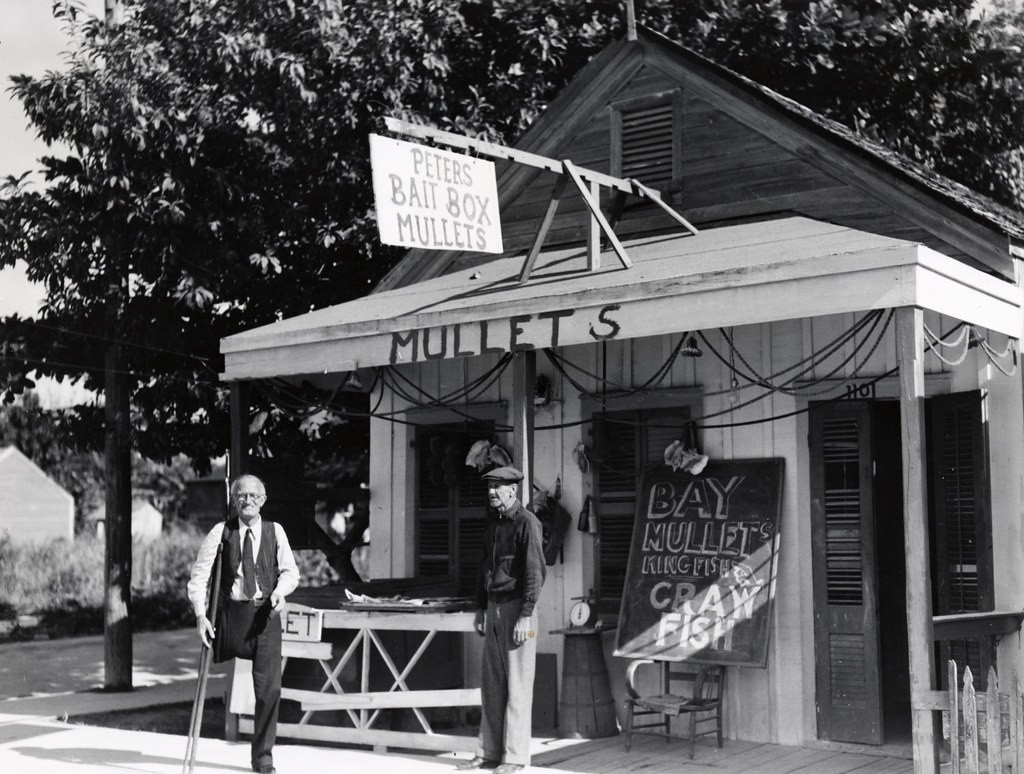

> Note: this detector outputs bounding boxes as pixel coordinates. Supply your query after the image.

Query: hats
[481,467,524,480]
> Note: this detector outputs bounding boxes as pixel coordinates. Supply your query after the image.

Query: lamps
[347,371,362,389]
[680,331,702,358]
[728,326,739,403]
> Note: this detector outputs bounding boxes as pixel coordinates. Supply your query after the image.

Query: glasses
[232,492,265,501]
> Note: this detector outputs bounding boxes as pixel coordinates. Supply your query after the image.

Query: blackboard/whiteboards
[611,457,787,670]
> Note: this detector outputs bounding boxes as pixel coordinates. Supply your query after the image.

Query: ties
[242,529,256,599]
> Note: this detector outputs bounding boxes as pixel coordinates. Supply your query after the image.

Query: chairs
[624,659,725,761]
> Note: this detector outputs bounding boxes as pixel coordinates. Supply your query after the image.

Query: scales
[568,588,601,632]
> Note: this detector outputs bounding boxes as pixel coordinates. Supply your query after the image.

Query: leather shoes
[252,765,277,774]
[492,763,526,774]
[457,756,501,770]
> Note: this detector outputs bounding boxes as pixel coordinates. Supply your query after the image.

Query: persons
[186,473,301,774]
[457,464,549,774]
